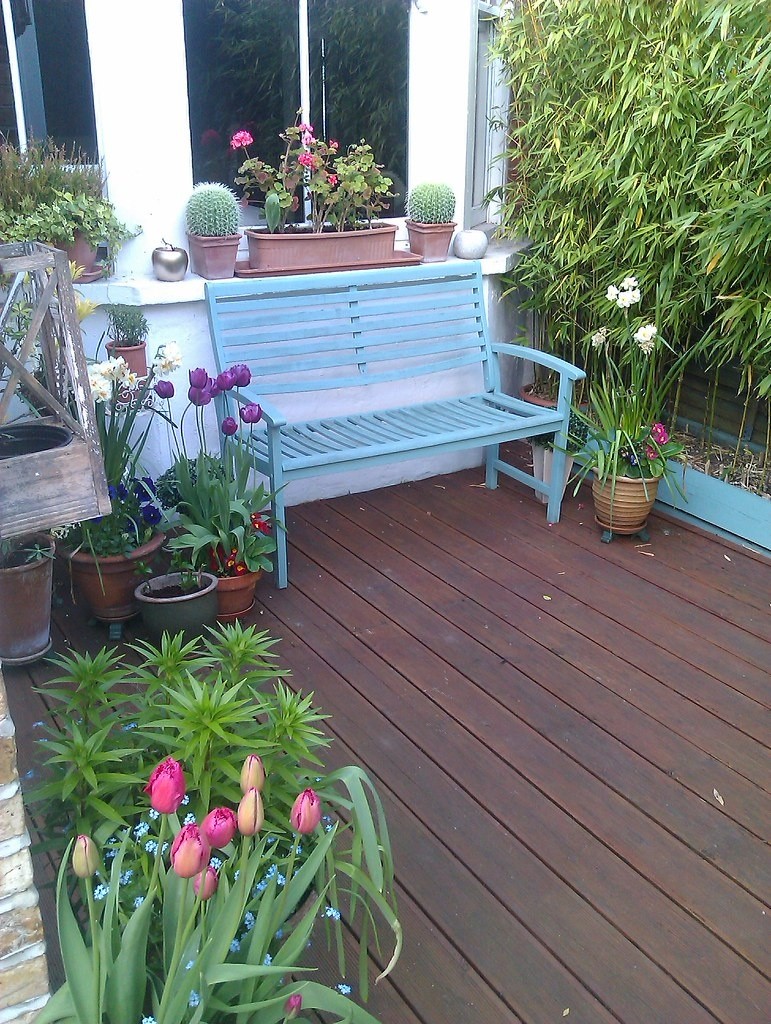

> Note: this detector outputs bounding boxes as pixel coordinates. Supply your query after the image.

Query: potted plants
[185,181,243,280]
[406,183,457,263]
[122,549,219,657]
[102,305,150,408]
[0,128,143,284]
[478,0,771,552]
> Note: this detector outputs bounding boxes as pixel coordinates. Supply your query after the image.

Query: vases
[591,466,663,535]
[219,568,263,623]
[55,531,167,623]
[0,534,58,666]
[244,222,400,269]
[0,425,74,461]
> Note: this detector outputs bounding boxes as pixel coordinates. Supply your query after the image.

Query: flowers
[548,276,689,538]
[28,754,405,1024]
[230,106,400,233]
[0,263,290,578]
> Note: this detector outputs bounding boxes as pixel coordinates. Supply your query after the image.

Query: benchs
[203,259,586,588]
